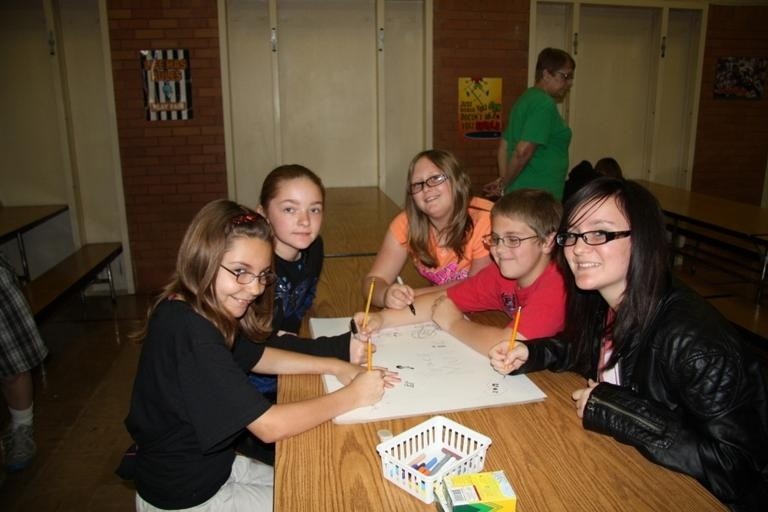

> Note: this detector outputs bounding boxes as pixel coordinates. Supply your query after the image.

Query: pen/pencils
[368,336,373,369]
[399,451,454,495]
[363,277,376,329]
[396,274,417,316]
[503,305,521,379]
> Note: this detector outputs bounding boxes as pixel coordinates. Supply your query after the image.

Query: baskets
[375,415,492,503]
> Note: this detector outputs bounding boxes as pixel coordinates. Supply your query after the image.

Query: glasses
[219,263,276,285]
[555,230,631,247]
[558,72,574,80]
[481,233,538,248]
[409,173,448,194]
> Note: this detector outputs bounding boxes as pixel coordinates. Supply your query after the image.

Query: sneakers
[0,420,37,470]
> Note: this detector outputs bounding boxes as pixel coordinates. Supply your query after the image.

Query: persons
[482,47,576,204]
[489,177,768,512]
[362,148,496,309]
[233,164,377,464]
[351,189,569,357]
[115,199,401,512]
[0,265,49,472]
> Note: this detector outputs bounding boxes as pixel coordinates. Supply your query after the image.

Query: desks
[630,179,767,304]
[272,255,731,512]
[1,203,68,280]
[322,187,405,256]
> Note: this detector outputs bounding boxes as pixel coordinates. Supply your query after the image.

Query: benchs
[706,295,768,340]
[672,264,735,299]
[19,241,123,387]
